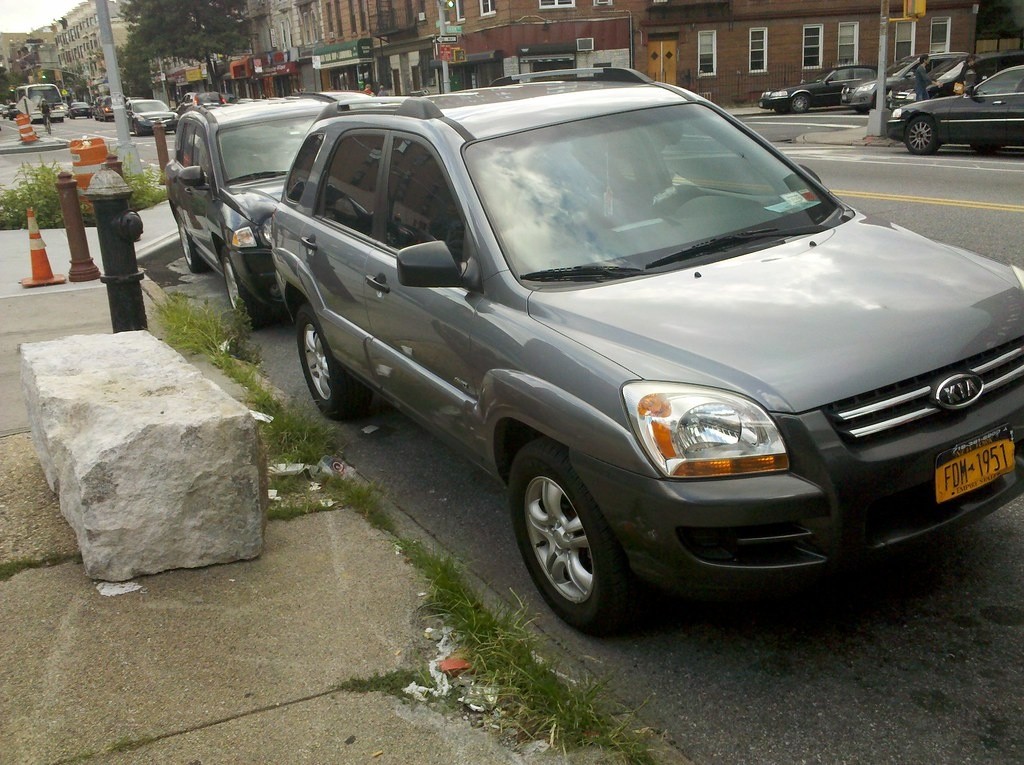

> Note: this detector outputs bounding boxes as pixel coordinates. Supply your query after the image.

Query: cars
[885,52,1024,114]
[0,75,266,136]
[885,64,1024,157]
[757,63,877,114]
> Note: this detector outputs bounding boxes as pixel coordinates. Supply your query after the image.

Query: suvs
[841,53,971,114]
[165,89,378,331]
[265,66,1024,637]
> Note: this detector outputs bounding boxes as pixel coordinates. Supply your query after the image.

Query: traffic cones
[21,206,66,287]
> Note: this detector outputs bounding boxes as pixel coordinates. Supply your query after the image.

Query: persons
[960,55,976,85]
[41,100,52,125]
[377,85,389,96]
[914,54,943,103]
[363,84,374,95]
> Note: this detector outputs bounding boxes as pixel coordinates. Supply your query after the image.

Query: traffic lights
[445,1,454,8]
